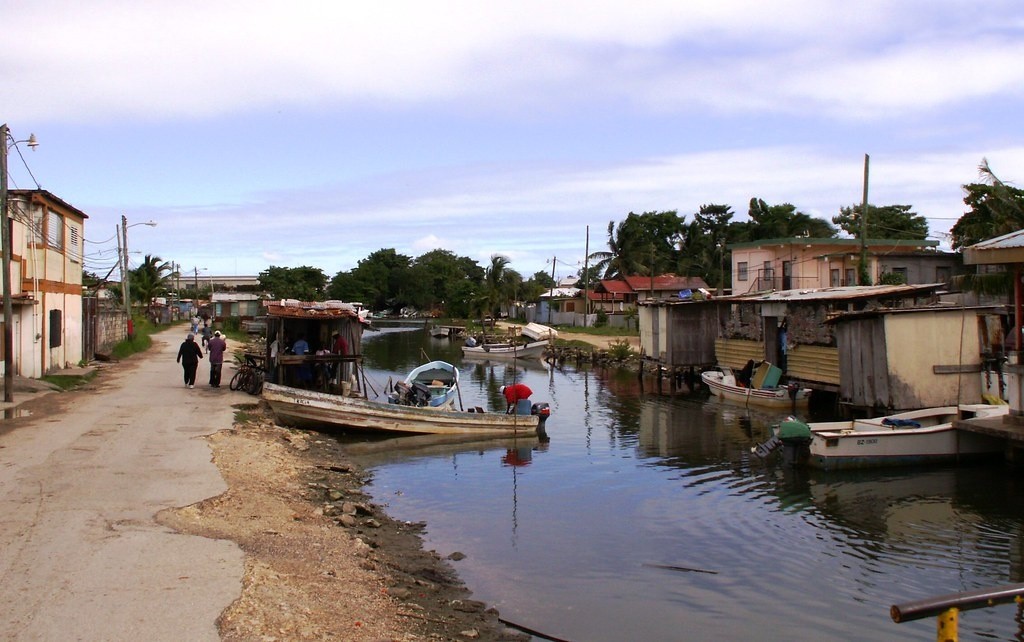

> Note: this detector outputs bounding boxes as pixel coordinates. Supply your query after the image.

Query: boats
[461,336,549,359]
[261,379,539,440]
[750,402,1010,459]
[700,364,813,407]
[429,326,449,337]
[388,359,460,408]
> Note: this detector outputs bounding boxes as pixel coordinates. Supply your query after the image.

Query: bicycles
[201,334,213,354]
[228,355,264,396]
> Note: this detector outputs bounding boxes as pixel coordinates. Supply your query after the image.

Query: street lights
[848,211,869,272]
[716,238,726,296]
[121,214,158,344]
[0,123,40,403]
[195,266,208,308]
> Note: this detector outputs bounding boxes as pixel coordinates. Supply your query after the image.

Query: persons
[499,383,533,415]
[177,333,203,388]
[270,331,349,383]
[208,330,226,388]
[190,311,213,350]
[738,359,765,389]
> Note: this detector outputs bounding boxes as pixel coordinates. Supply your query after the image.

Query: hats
[499,386,505,395]
[332,330,338,336]
[215,330,221,335]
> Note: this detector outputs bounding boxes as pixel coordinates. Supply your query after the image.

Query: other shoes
[185,384,188,387]
[190,385,194,388]
[211,384,215,387]
[215,386,220,388]
